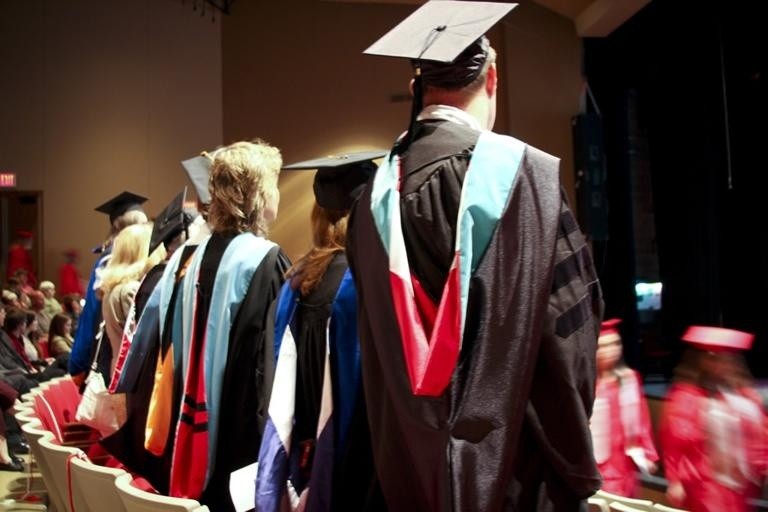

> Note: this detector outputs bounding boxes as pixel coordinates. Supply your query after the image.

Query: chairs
[8,366,226,512]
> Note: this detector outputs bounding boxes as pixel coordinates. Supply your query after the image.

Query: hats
[94,191,150,226]
[149,186,199,254]
[362,0,520,89]
[179,146,219,205]
[281,150,386,210]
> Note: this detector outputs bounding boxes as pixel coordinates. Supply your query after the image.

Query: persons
[1,271,86,473]
[5,230,39,290]
[68,140,390,510]
[344,0,602,512]
[59,249,86,300]
[660,326,768,512]
[591,317,659,501]
